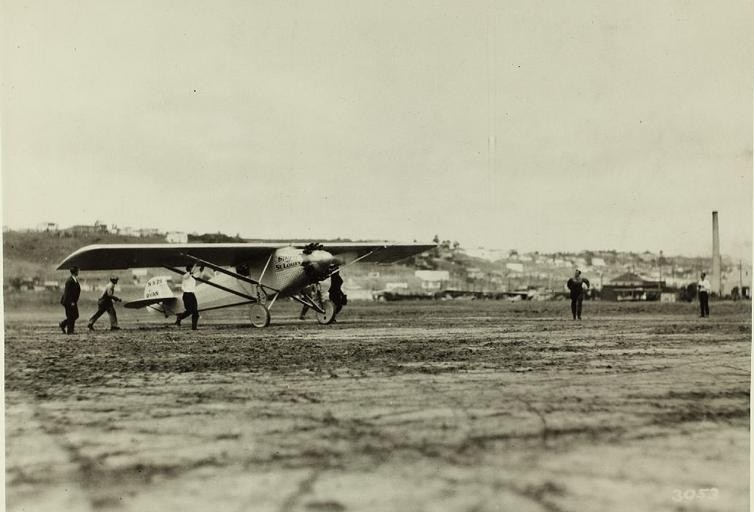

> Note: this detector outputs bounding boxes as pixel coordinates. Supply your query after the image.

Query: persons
[87,276,122,331]
[567,270,590,320]
[696,273,711,319]
[176,261,205,330]
[59,265,81,335]
[328,267,346,324]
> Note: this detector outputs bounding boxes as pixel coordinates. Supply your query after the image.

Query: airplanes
[56,242,439,328]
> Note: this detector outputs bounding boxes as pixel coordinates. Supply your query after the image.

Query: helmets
[110,276,118,284]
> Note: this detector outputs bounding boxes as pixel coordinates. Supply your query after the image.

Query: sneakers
[59,321,78,335]
[111,326,120,330]
[177,313,181,326]
[88,323,95,331]
